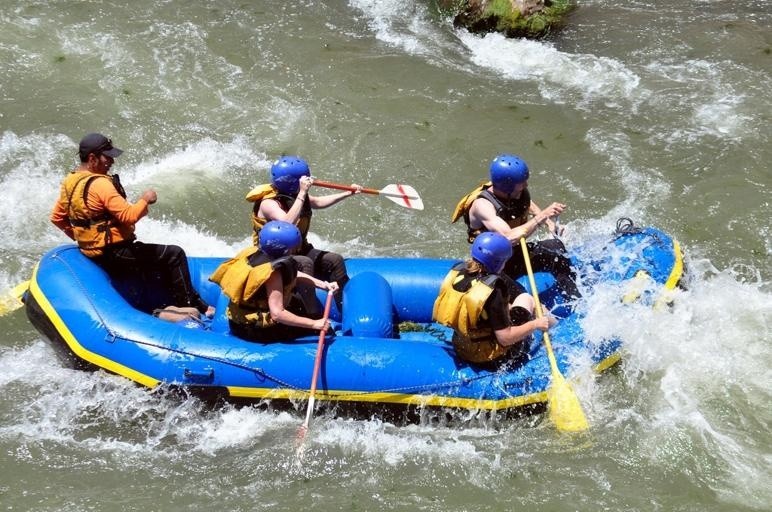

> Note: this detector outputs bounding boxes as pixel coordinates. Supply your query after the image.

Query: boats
[20,227,685,426]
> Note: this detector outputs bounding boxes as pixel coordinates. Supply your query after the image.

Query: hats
[79,134,124,158]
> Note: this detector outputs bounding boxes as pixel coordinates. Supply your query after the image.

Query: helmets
[490,154,529,193]
[471,232,513,275]
[270,156,311,193]
[259,220,302,254]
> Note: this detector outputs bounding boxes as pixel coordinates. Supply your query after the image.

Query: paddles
[520,229,597,449]
[1,277,31,316]
[314,181,424,210]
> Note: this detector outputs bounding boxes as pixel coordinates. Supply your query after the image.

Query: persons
[50,133,216,323]
[226,221,339,344]
[251,156,363,314]
[463,154,581,301]
[452,233,559,376]
